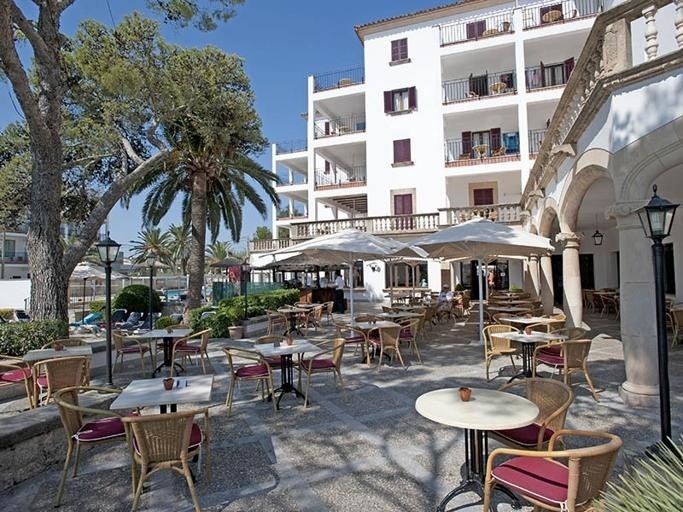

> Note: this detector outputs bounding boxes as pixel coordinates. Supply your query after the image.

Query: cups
[163,378,174,390]
[287,336,293,345]
[368,320,371,326]
[526,329,531,335]
[176,378,187,389]
[458,388,472,401]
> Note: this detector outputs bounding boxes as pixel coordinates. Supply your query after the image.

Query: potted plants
[225,305,245,339]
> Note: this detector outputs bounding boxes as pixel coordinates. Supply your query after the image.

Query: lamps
[591,213,603,245]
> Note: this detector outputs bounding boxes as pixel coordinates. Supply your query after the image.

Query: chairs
[497,22,510,33]
[485,378,575,475]
[464,92,480,98]
[483,429,623,512]
[665,298,683,352]
[121,407,211,512]
[54,386,136,508]
[557,9,577,20]
[491,146,506,156]
[532,339,600,403]
[583,288,620,321]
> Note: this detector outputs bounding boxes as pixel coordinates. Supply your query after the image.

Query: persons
[438,284,454,302]
[320,271,330,288]
[487,268,496,295]
[329,269,345,314]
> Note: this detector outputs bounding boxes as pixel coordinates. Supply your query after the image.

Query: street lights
[97,230,121,390]
[143,249,158,332]
[240,257,251,319]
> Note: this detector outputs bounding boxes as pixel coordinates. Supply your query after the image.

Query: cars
[0,309,29,327]
[68,311,105,329]
[152,286,214,302]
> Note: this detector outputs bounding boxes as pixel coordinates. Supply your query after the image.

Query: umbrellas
[440,255,528,301]
[134,260,171,276]
[208,258,242,285]
[413,217,555,342]
[250,228,431,337]
